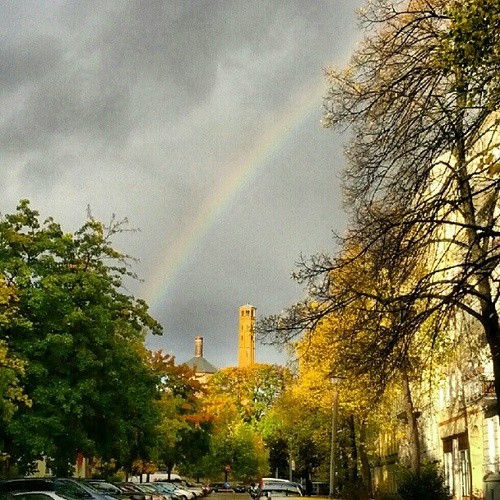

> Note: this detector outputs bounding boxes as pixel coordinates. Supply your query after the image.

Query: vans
[207,482,230,494]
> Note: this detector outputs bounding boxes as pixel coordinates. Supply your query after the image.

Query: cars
[260,477,304,497]
[233,482,245,493]
[0,476,212,500]
